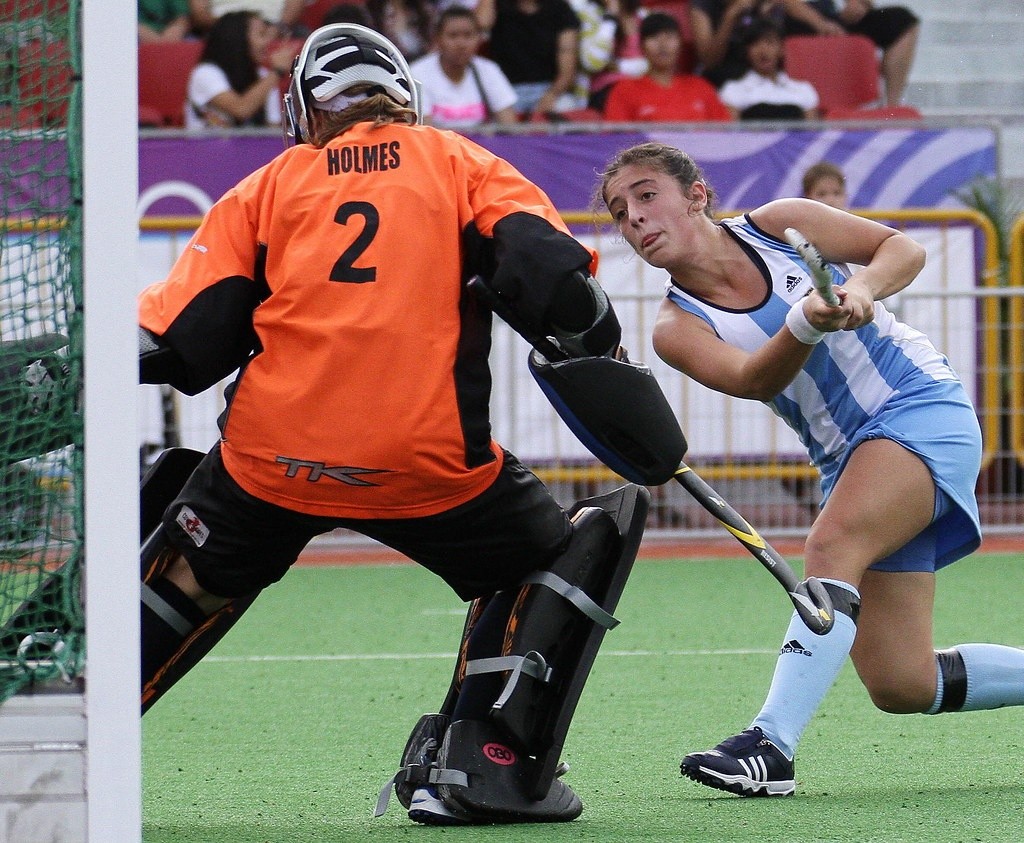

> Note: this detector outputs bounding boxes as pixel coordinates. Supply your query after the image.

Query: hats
[209,0,283,24]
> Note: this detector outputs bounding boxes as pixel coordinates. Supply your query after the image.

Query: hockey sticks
[782,227,841,310]
[673,459,835,637]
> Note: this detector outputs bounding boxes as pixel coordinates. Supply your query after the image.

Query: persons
[591,144,1024,795]
[0,22,687,824]
[137,0,921,128]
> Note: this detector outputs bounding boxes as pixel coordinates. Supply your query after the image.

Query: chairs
[0,32,924,129]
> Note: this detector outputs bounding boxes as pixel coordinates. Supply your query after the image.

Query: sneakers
[680,726,799,799]
[406,760,464,823]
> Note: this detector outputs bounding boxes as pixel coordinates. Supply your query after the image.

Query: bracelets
[785,296,826,345]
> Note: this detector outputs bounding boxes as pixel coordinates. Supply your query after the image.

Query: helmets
[284,22,423,145]
